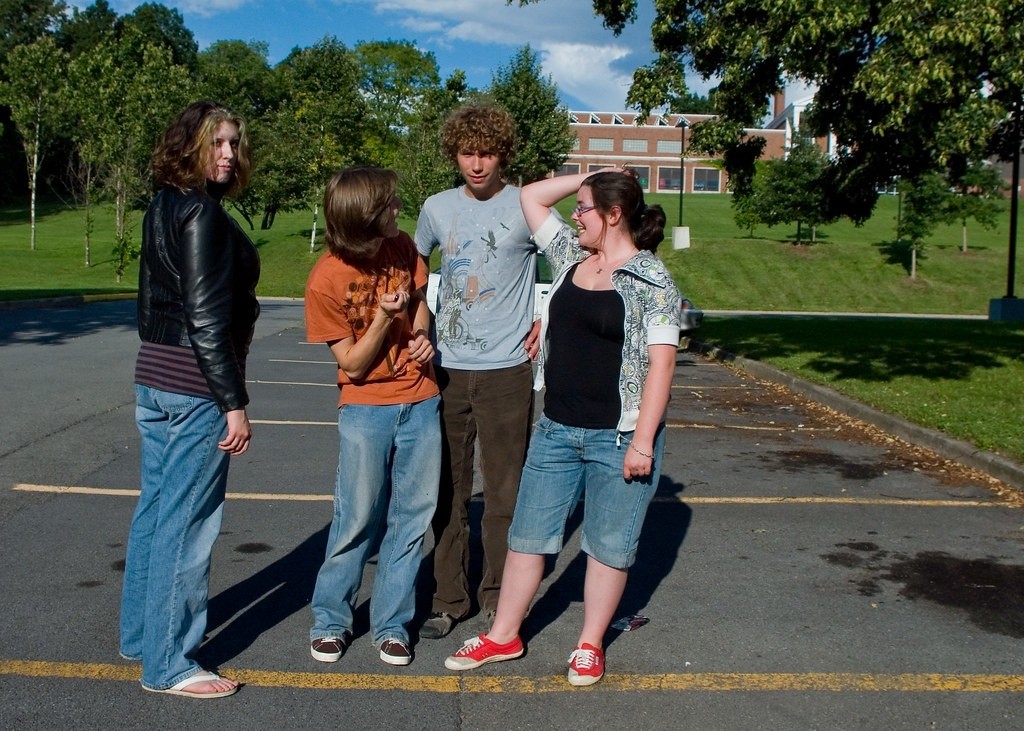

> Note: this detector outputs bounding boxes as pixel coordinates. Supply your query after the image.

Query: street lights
[674,121,688,227]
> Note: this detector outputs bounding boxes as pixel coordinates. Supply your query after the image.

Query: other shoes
[415,614,454,638]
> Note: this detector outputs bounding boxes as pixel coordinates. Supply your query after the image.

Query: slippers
[142,671,238,698]
[119,651,141,660]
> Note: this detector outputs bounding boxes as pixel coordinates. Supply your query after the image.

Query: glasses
[571,205,606,216]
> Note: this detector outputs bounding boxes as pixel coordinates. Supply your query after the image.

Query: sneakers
[310,637,345,663]
[566,644,605,686]
[444,632,524,672]
[377,638,412,665]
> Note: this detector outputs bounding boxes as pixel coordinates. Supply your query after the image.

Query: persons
[445,166,682,688]
[412,101,569,638]
[303,164,442,667]
[119,99,261,699]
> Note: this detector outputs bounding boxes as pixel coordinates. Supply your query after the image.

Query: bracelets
[630,441,654,459]
